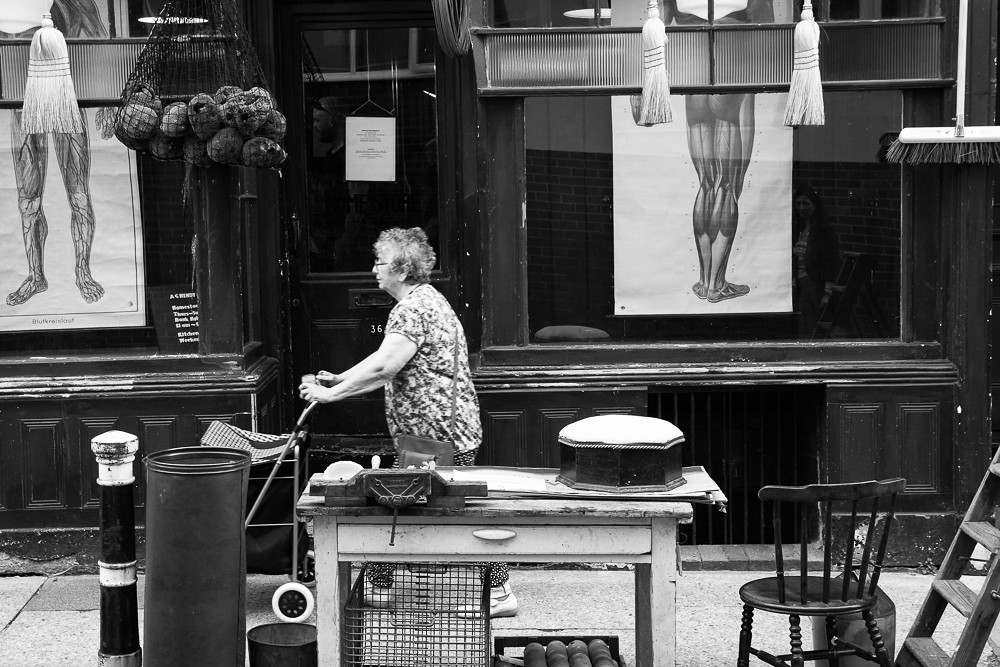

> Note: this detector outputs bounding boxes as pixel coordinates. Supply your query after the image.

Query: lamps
[561,1,612,20]
[676,0,750,22]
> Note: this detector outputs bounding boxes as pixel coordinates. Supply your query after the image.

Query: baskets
[344,562,491,667]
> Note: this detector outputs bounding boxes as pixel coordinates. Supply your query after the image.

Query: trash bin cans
[142,446,252,667]
[246,621,318,667]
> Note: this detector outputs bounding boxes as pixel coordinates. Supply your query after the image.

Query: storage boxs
[558,416,689,493]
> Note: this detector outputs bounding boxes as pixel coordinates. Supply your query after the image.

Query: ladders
[893,443,1000,667]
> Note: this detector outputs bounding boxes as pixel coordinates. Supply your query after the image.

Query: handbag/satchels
[398,434,454,468]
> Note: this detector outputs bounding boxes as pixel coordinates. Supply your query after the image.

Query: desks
[296,473,697,667]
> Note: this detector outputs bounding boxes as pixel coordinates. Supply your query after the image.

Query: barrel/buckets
[247,623,317,667]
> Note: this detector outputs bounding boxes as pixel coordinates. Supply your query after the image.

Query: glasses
[374,259,390,268]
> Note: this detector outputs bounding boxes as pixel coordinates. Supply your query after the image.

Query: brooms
[634,0,678,129]
[19,0,89,138]
[883,0,1000,170]
[779,0,829,129]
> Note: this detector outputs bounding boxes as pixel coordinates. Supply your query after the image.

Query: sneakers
[454,583,518,619]
[361,586,390,608]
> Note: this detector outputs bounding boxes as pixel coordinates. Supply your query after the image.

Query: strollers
[197,370,328,623]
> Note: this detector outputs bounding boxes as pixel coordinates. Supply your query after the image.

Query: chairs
[736,476,907,667]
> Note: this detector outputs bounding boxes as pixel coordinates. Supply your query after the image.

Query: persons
[300,227,517,620]
[792,191,838,338]
[313,96,370,272]
